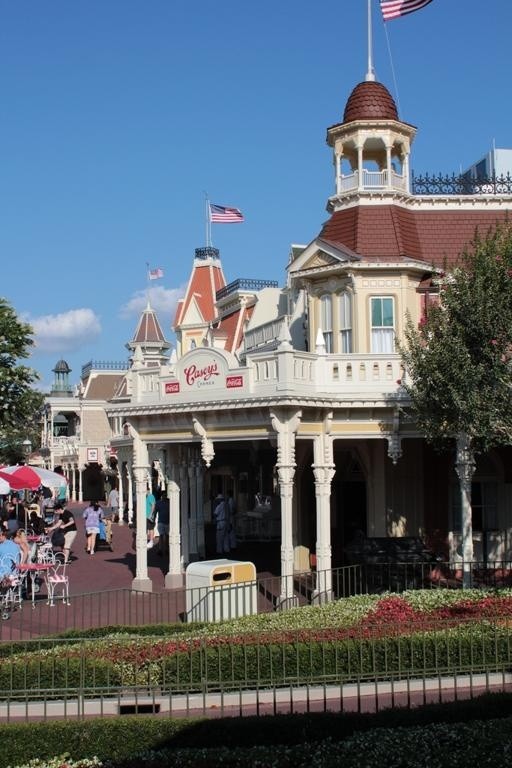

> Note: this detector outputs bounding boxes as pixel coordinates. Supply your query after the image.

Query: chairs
[0,527,70,621]
[341,536,438,592]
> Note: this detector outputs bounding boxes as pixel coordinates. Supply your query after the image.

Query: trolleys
[85,518,114,553]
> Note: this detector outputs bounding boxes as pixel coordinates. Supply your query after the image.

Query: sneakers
[86,547,95,554]
[146,541,153,548]
[58,558,72,566]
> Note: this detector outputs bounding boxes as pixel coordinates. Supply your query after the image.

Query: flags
[209,203,244,223]
[148,267,164,280]
[380,0,433,22]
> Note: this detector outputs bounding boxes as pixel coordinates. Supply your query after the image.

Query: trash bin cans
[186,559,257,622]
[26,503,44,520]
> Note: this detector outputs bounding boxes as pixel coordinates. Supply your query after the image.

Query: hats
[214,493,224,499]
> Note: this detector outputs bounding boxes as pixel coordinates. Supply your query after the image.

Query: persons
[213,489,237,558]
[0,486,78,593]
[82,498,104,555]
[253,492,266,507]
[146,487,169,555]
[107,484,119,523]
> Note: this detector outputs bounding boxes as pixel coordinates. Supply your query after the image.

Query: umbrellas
[0,463,70,532]
